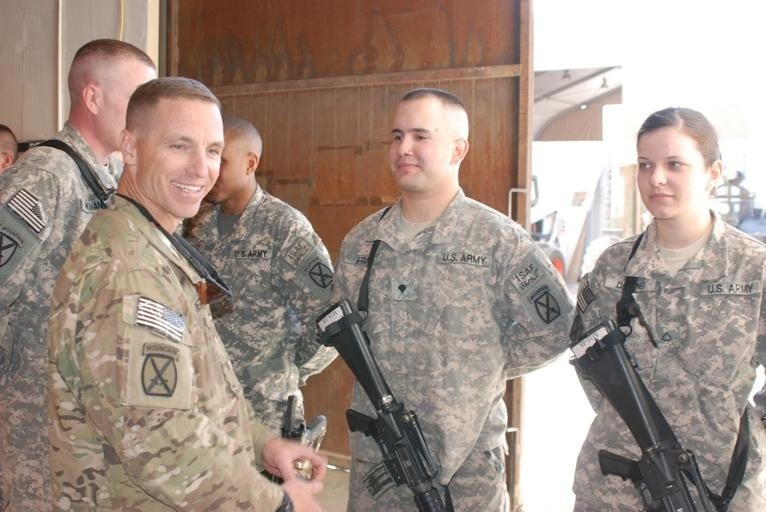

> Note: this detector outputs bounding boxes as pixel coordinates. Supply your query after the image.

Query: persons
[334,89,574,512]
[572,107,766,512]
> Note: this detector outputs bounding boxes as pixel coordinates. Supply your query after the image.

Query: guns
[316,298,445,512]
[569,318,716,511]
